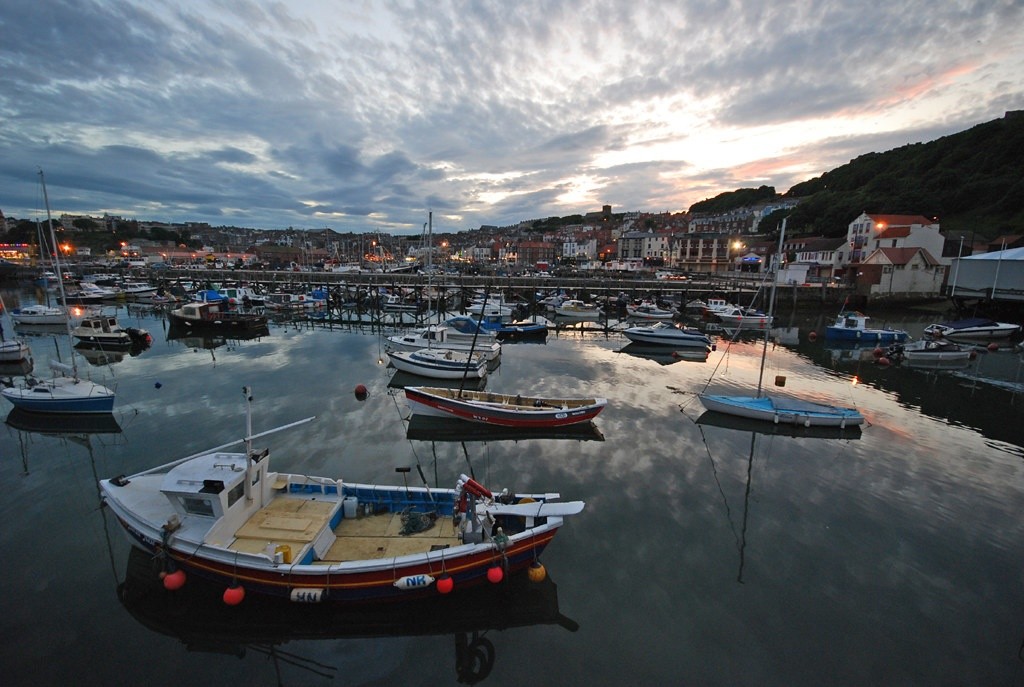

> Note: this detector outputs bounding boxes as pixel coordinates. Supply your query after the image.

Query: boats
[922,317,1022,348]
[99,385,585,612]
[885,341,970,363]
[824,309,906,346]
[621,321,712,346]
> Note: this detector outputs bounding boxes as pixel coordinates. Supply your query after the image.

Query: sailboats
[697,214,865,428]
[0,207,772,379]
[402,274,607,433]
[0,167,120,419]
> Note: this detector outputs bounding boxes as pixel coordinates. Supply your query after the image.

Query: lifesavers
[460,473,495,501]
[457,480,485,500]
